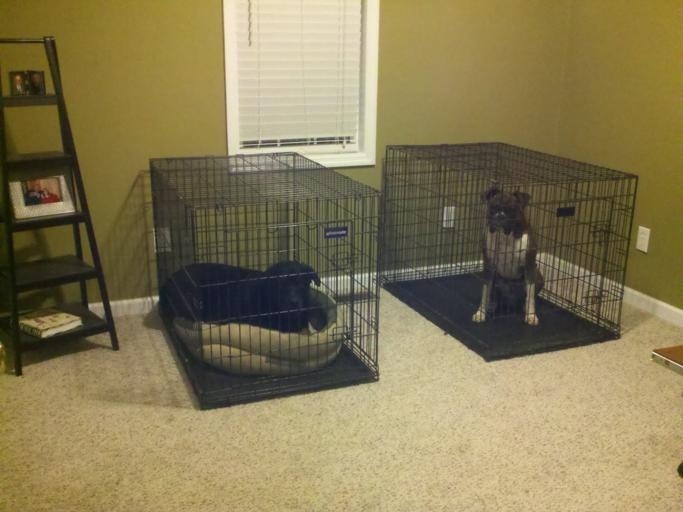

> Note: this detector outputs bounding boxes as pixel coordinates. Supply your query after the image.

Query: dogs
[471,186,545,327]
[159,260,321,333]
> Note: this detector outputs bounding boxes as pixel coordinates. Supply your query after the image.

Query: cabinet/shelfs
[0,34,118,376]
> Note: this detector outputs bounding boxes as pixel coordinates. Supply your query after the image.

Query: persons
[27,185,61,205]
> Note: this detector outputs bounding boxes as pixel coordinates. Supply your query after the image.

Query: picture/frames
[8,175,76,221]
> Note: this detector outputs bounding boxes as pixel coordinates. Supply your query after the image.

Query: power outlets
[154,228,171,253]
[636,225,651,254]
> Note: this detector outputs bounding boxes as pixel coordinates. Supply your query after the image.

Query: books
[10,310,83,339]
